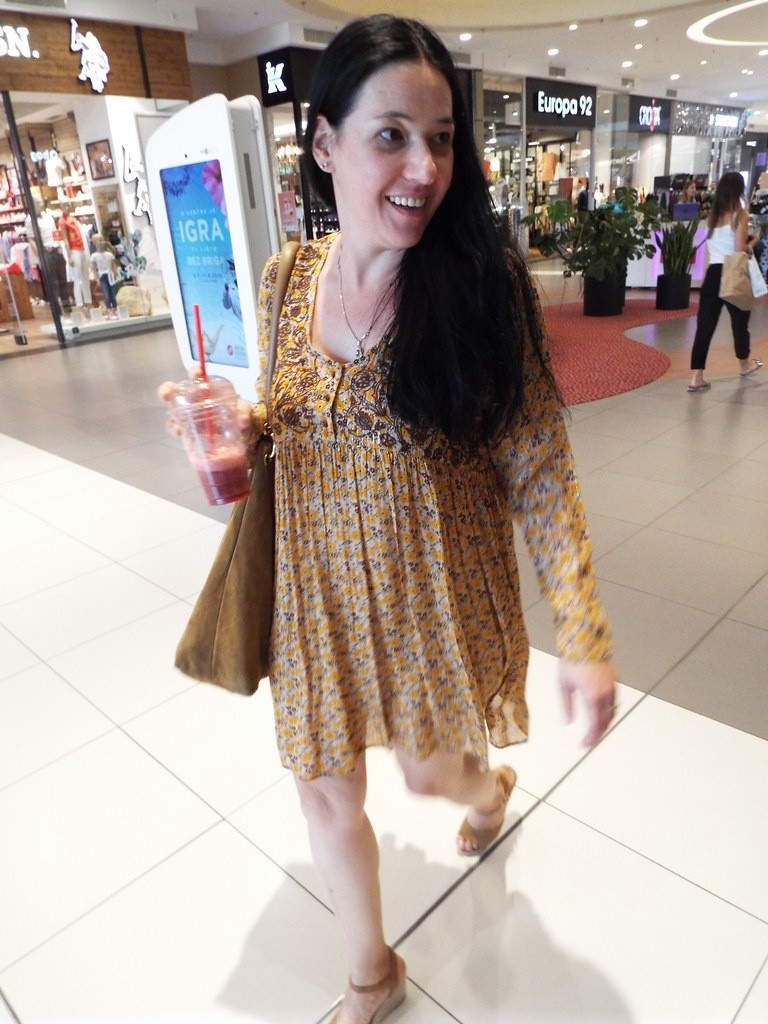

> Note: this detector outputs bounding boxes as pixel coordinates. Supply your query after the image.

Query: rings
[607,704,619,712]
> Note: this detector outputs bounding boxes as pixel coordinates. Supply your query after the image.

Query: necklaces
[335,234,400,358]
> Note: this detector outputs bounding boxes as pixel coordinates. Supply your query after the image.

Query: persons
[750,171,768,283]
[58,197,95,323]
[686,172,763,391]
[108,234,124,256]
[153,11,618,1024]
[44,157,67,187]
[91,232,122,321]
[616,176,639,208]
[677,180,697,204]
[594,183,607,210]
[576,177,598,224]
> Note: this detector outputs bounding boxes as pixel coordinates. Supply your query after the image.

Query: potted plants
[520,187,665,315]
[655,212,707,310]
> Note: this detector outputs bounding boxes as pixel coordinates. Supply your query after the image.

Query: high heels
[331,946,406,1024]
[455,766,517,851]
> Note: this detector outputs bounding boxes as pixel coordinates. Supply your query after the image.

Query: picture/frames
[86,140,115,180]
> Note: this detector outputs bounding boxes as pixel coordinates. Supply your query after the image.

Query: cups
[173,374,255,505]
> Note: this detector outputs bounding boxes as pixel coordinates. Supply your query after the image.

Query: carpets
[542,299,700,406]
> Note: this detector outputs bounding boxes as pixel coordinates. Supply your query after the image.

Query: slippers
[739,359,763,376]
[687,380,711,392]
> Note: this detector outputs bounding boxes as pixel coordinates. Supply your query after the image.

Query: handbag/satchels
[718,251,756,311]
[174,434,276,696]
[747,244,768,298]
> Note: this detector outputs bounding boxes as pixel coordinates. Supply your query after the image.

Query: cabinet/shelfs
[50,198,95,217]
[0,208,26,225]
